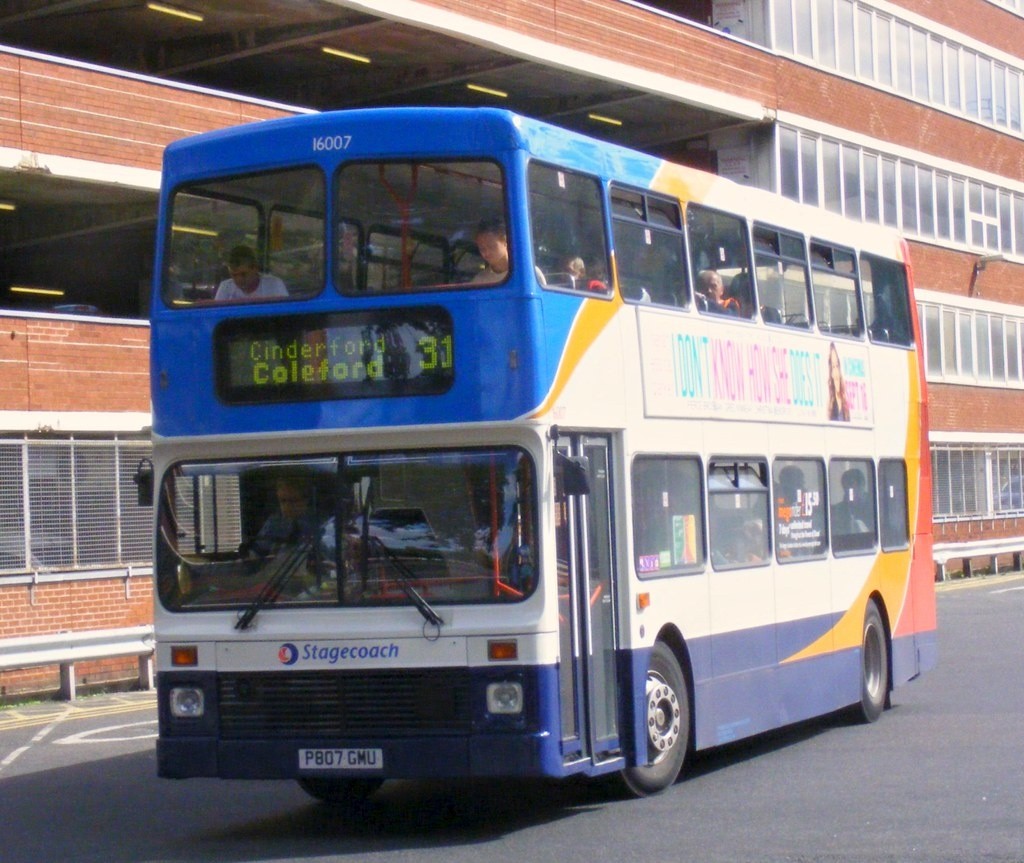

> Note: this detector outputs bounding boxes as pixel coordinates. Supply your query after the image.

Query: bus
[134,108,938,805]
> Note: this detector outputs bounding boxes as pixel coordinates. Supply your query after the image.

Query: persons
[557,253,607,294]
[470,218,547,288]
[831,468,874,525]
[730,273,769,321]
[214,245,289,300]
[779,465,819,511]
[254,475,319,553]
[695,269,739,317]
[721,528,764,563]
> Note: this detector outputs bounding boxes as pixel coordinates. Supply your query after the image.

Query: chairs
[536,259,896,342]
